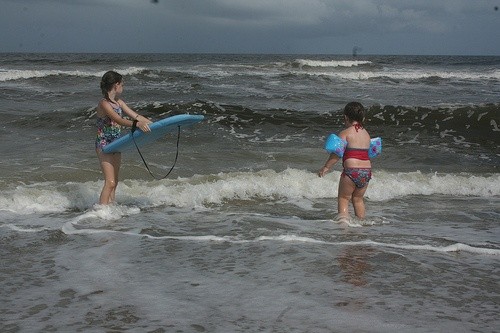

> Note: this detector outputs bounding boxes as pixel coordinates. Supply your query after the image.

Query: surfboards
[100,113,205,156]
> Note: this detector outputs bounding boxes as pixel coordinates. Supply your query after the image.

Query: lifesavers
[367,138,381,161]
[324,134,348,158]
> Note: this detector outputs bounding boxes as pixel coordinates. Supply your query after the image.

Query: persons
[319,102,375,225]
[93,70,154,204]
